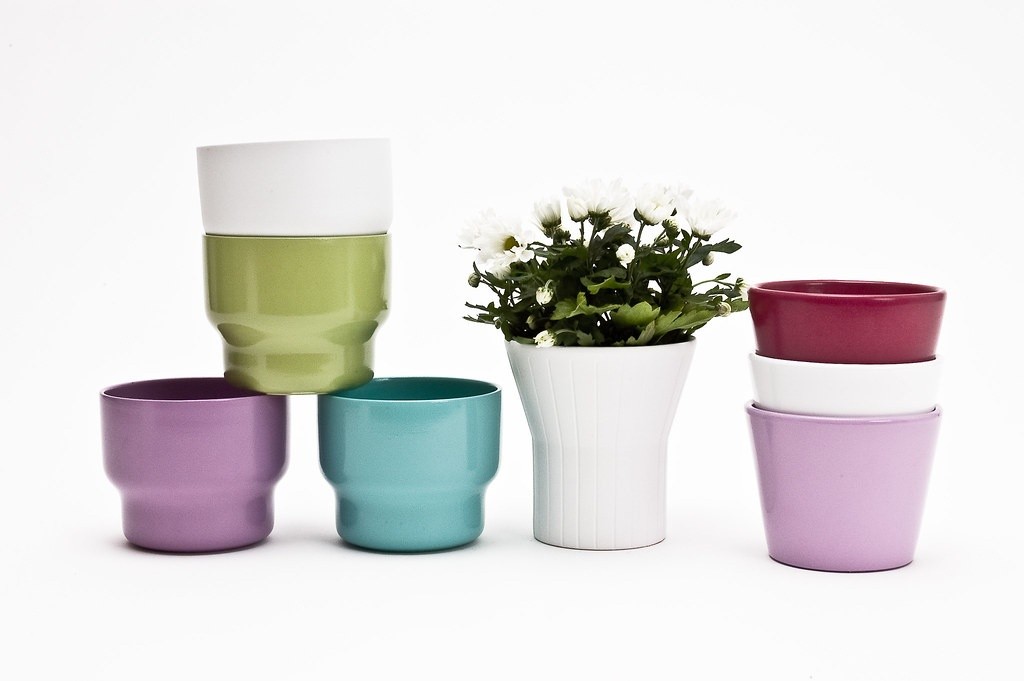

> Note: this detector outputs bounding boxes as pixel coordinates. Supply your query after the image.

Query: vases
[744,400,944,573]
[318,377,501,552]
[747,279,947,364]
[195,135,395,238]
[749,348,944,418]
[201,234,393,396]
[501,334,699,546]
[100,377,292,553]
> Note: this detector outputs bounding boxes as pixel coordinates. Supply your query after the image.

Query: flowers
[459,170,753,348]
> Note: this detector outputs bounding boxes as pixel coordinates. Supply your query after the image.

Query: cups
[746,349,944,415]
[98,377,287,553]
[317,377,502,553]
[206,233,393,396]
[746,400,945,572]
[746,279,947,366]
[194,139,395,239]
